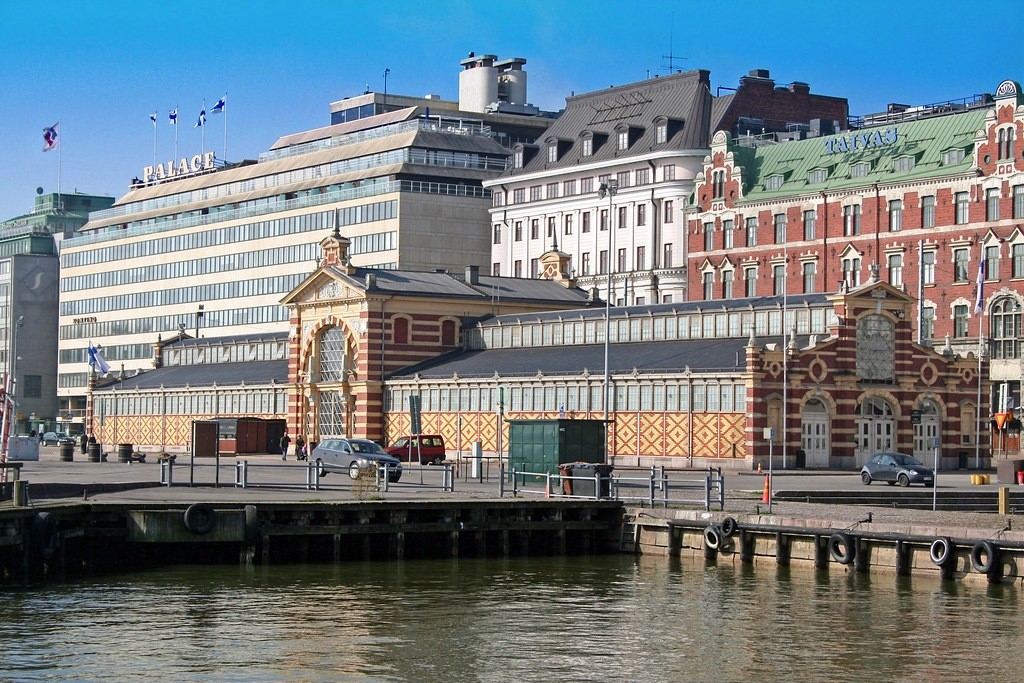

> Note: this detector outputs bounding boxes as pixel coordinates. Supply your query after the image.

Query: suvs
[42,431,76,447]
[311,438,402,482]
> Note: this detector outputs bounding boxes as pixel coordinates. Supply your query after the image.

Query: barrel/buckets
[118,444,133,463]
[59,442,74,461]
[970,474,991,485]
[88,443,100,462]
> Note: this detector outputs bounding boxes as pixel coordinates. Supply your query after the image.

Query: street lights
[10,314,25,435]
[598,177,626,498]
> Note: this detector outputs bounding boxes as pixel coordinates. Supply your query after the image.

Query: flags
[41,120,59,152]
[87,341,111,374]
[210,95,225,113]
[193,107,206,127]
[148,110,157,125]
[169,108,178,125]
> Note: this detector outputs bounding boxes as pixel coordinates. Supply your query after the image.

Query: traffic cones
[755,473,778,505]
[756,463,763,473]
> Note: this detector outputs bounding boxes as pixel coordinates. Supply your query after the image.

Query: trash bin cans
[796,449,805,469]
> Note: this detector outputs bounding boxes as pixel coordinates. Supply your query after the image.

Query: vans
[384,434,446,465]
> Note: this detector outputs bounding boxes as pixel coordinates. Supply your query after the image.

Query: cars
[860,452,935,487]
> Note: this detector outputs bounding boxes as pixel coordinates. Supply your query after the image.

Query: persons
[279,432,291,462]
[80,431,88,454]
[88,434,96,443]
[296,434,305,460]
[38,431,44,445]
[29,428,36,437]
